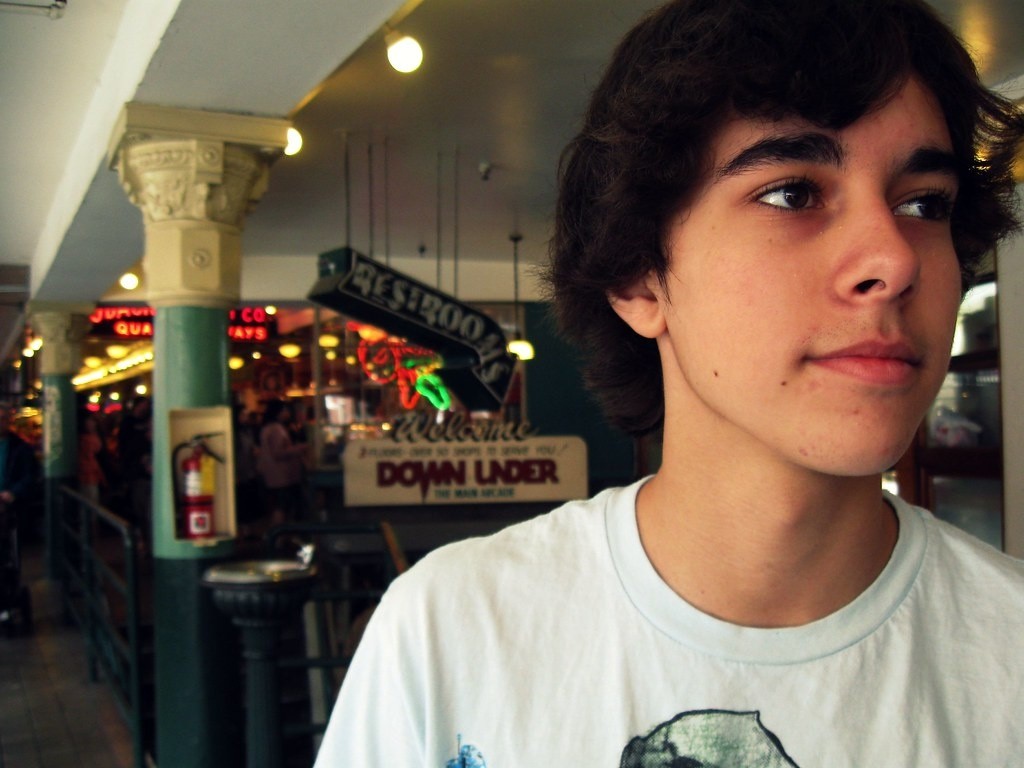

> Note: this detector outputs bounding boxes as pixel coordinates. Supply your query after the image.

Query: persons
[0,359,318,634]
[312,0,1024,768]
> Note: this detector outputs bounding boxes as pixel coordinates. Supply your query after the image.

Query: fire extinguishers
[171,429,226,538]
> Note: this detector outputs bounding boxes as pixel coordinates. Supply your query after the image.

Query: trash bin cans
[199,559,317,768]
